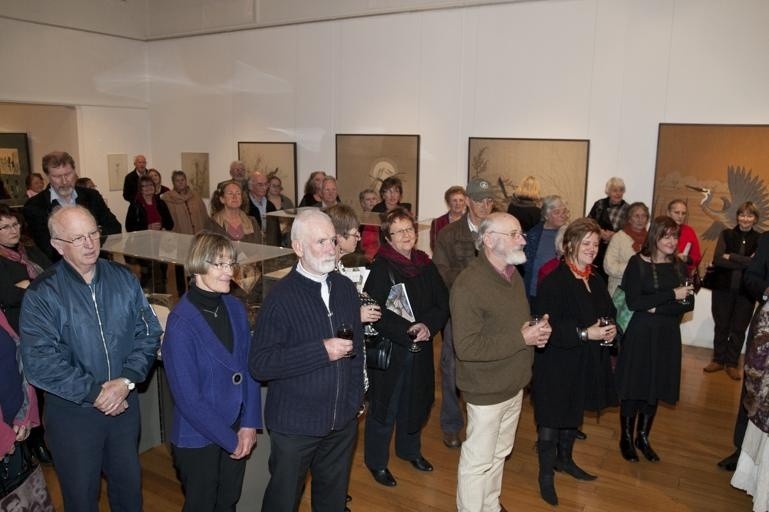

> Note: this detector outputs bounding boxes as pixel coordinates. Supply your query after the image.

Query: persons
[249,207,364,511]
[361,208,450,487]
[1,207,53,512]
[703,202,768,511]
[160,232,264,512]
[21,202,163,512]
[431,177,701,511]
[0,152,449,294]
[319,205,381,323]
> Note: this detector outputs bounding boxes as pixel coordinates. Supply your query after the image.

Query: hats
[466,178,497,202]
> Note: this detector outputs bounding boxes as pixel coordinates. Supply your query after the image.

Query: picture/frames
[237,141,298,208]
[334,133,420,221]
[467,137,590,225]
[0,133,31,199]
[651,123,768,287]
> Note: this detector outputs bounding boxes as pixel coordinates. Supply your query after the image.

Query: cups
[528,313,547,330]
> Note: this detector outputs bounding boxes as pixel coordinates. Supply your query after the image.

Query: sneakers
[703,360,724,372]
[726,367,741,381]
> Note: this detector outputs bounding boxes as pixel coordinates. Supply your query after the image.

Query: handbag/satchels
[703,268,715,289]
[0,464,54,512]
[612,284,634,335]
[124,238,151,267]
[364,333,394,371]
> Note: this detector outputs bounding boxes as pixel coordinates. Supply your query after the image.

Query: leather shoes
[717,449,740,471]
[36,443,52,464]
[442,431,461,448]
[367,465,397,485]
[576,429,586,439]
[411,457,434,470]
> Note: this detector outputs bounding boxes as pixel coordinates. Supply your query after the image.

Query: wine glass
[406,321,425,354]
[363,297,381,337]
[336,321,357,359]
[598,315,616,348]
[679,280,694,305]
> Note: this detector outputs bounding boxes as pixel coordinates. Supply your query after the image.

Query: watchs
[119,377,135,390]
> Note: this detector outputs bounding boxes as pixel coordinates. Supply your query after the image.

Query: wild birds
[684,164,769,241]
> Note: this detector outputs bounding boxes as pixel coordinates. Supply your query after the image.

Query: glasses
[205,260,239,271]
[390,227,416,237]
[54,225,102,247]
[344,232,362,240]
[1,223,22,231]
[489,230,526,240]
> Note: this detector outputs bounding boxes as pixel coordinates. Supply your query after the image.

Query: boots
[555,431,597,480]
[619,409,638,462]
[636,413,660,461]
[536,437,558,506]
